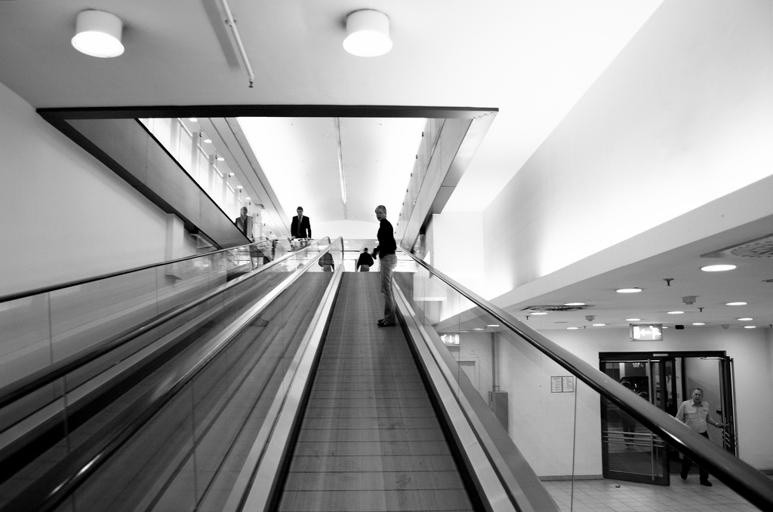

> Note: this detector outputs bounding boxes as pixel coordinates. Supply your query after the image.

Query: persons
[290,206,310,239]
[673,389,729,487]
[355,247,374,273]
[373,202,400,327]
[233,206,254,243]
[619,408,636,450]
[318,252,337,272]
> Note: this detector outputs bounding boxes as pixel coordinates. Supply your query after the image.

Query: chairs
[377,318,397,328]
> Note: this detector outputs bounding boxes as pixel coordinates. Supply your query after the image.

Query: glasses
[192,118,252,203]
[71,9,126,58]
[341,8,392,59]
